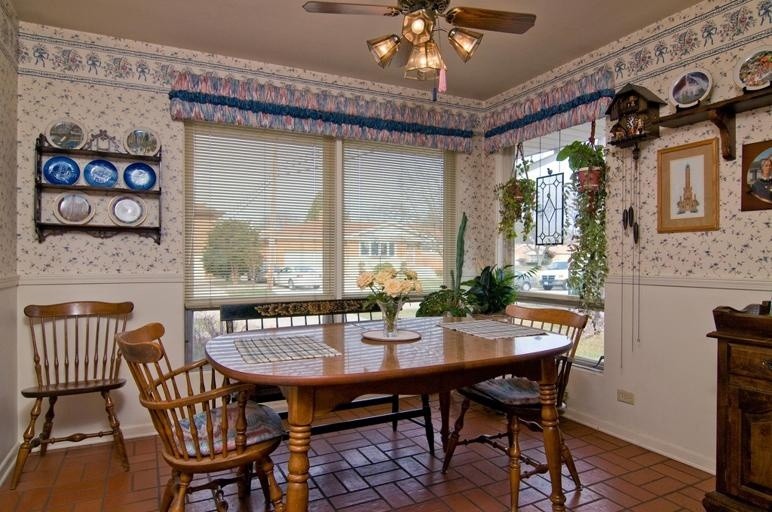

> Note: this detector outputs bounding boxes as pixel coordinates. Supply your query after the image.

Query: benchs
[219,298,436,455]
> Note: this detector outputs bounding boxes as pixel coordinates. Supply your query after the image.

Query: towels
[233,334,342,365]
[436,319,546,341]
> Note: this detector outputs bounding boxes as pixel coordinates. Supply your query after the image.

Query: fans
[302,0,537,35]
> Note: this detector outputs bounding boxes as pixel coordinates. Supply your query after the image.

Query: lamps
[366,8,483,81]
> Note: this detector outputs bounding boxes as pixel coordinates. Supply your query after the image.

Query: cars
[275,266,322,289]
[247,265,279,283]
[514,270,535,292]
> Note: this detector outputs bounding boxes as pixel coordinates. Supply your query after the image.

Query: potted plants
[556,141,611,336]
[492,160,540,241]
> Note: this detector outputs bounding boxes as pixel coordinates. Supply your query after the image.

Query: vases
[375,300,407,338]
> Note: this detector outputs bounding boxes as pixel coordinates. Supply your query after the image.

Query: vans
[540,254,585,291]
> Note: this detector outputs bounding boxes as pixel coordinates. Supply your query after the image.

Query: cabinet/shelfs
[33,132,163,245]
[701,299,771,511]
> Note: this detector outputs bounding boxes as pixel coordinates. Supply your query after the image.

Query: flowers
[357,268,422,329]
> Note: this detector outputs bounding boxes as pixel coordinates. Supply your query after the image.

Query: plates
[360,328,422,343]
[84,160,119,187]
[53,191,96,225]
[123,162,156,191]
[42,156,80,185]
[106,194,147,226]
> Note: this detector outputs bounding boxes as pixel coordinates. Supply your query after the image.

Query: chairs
[11,300,135,491]
[116,322,286,512]
[441,303,588,512]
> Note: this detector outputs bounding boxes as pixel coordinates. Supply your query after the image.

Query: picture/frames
[741,140,772,211]
[656,136,720,234]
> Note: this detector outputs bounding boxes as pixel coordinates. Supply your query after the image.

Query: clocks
[604,82,668,150]
